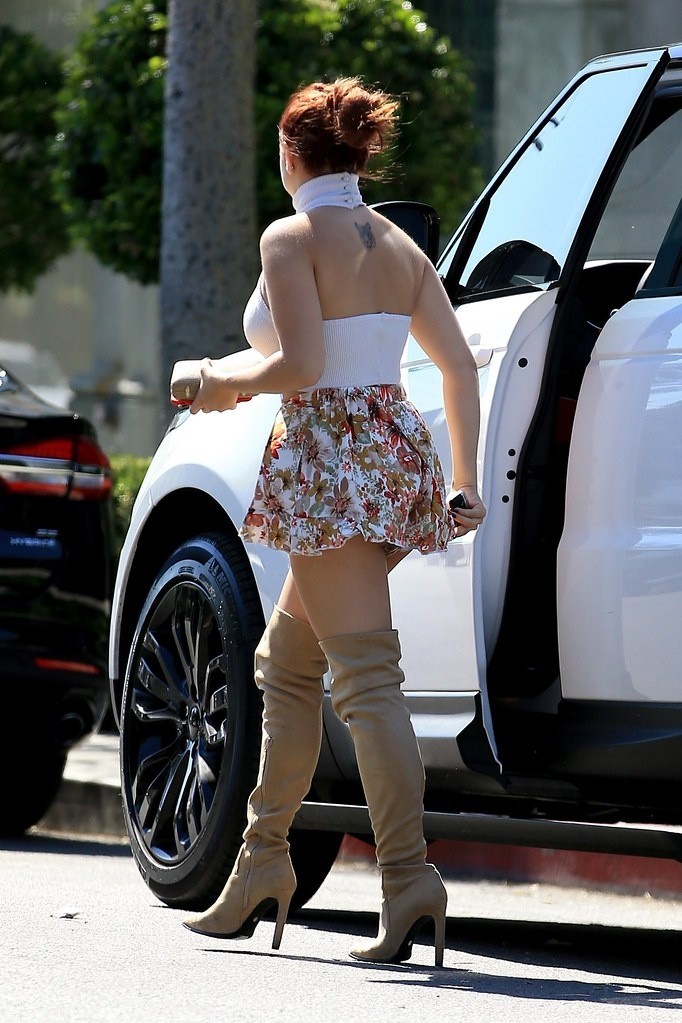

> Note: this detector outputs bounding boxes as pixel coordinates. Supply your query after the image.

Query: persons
[182,78,489,969]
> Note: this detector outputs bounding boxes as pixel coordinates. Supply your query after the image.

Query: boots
[182,600,327,950]
[319,630,448,967]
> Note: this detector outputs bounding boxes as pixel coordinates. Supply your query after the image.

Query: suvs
[106,41,682,920]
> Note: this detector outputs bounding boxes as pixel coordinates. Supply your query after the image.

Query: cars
[0,360,116,836]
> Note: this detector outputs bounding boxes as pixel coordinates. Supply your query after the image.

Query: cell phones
[447,491,471,527]
[170,395,250,405]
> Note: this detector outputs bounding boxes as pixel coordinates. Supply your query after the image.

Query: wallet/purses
[170,360,258,407]
